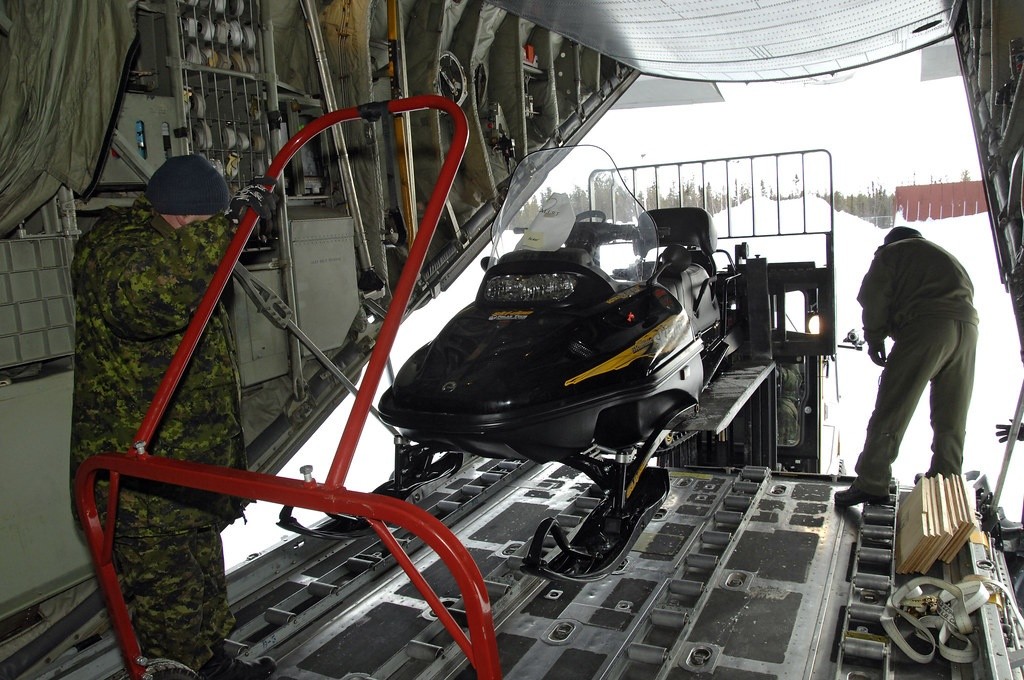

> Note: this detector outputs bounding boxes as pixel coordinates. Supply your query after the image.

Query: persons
[69,154,283,680]
[834,226,980,506]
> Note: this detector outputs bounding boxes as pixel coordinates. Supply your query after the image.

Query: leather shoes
[200,640,278,680]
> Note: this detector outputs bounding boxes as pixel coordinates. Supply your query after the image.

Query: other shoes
[833,481,890,508]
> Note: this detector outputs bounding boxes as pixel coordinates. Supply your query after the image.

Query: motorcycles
[275,143,751,584]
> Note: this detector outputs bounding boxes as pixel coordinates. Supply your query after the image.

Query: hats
[144,153,230,215]
[885,227,922,244]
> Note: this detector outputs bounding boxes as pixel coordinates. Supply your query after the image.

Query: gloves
[228,174,279,221]
